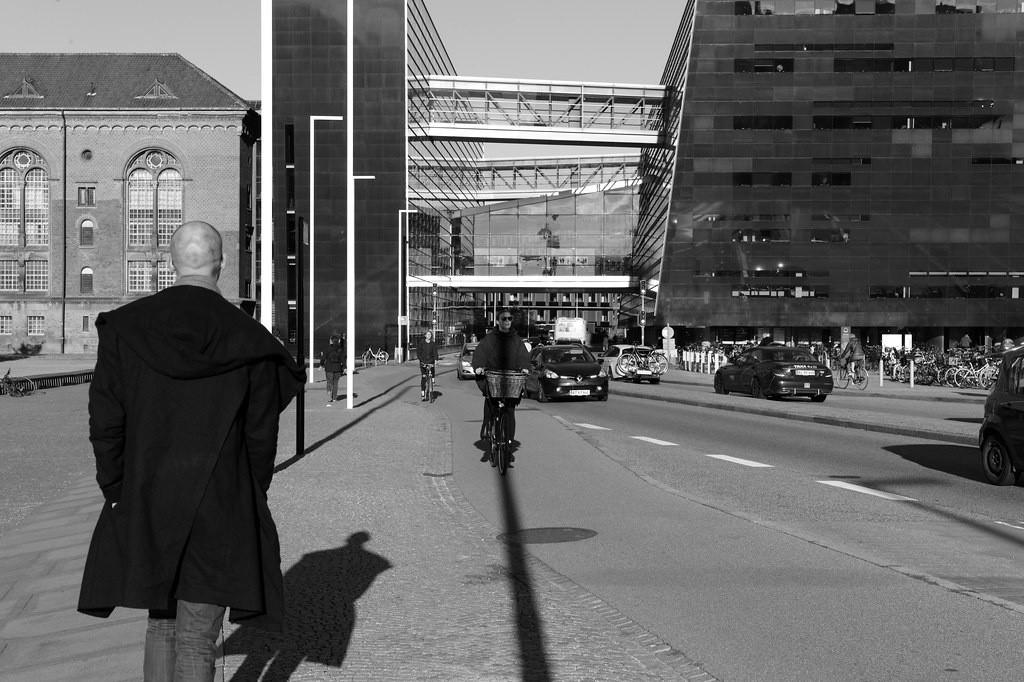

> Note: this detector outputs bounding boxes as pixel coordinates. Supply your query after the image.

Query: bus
[518,317,587,346]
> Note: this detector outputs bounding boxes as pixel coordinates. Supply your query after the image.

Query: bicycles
[419,361,439,403]
[480,367,531,475]
[616,341,669,376]
[0,367,36,398]
[833,355,869,391]
[361,343,389,362]
[861,341,1002,391]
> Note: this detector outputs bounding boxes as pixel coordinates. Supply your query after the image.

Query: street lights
[308,116,344,383]
[397,210,419,364]
[351,176,377,373]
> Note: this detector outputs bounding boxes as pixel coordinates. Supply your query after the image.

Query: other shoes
[849,371,856,376]
[431,377,435,383]
[421,390,425,399]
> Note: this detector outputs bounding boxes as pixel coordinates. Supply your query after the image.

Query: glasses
[499,316,514,321]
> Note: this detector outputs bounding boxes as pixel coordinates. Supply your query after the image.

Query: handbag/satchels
[847,357,852,363]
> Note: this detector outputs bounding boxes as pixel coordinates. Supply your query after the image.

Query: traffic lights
[639,310,646,326]
[640,280,647,294]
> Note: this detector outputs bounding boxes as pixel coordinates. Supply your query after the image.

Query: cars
[714,346,835,403]
[979,344,1024,487]
[597,344,661,385]
[521,342,609,404]
[455,342,480,380]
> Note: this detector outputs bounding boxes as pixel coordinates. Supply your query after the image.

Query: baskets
[486,374,527,398]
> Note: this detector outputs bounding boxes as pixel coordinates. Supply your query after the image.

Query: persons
[319,334,343,402]
[339,333,346,349]
[840,334,865,385]
[472,309,531,462]
[602,335,608,351]
[273,328,285,347]
[959,334,972,347]
[77,221,298,682]
[417,331,438,401]
[521,337,532,352]
[461,333,478,346]
[758,333,774,346]
